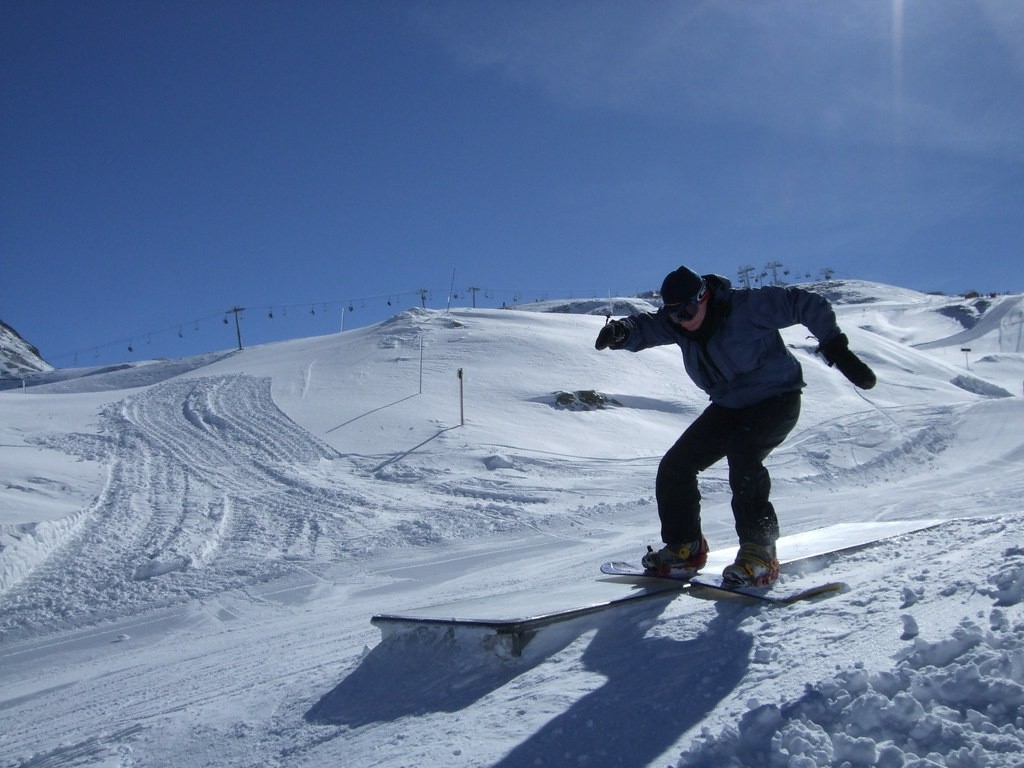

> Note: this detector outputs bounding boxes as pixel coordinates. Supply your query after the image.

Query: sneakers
[641,537,709,575]
[723,544,780,587]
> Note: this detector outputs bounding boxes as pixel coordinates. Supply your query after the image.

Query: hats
[659,265,708,305]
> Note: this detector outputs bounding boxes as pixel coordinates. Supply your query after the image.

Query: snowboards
[599,559,841,602]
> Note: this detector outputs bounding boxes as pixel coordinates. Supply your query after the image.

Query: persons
[594,266,877,587]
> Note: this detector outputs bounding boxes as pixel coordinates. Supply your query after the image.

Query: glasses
[669,278,707,323]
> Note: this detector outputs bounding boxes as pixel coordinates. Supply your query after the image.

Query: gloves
[834,348,877,389]
[595,322,626,351]
